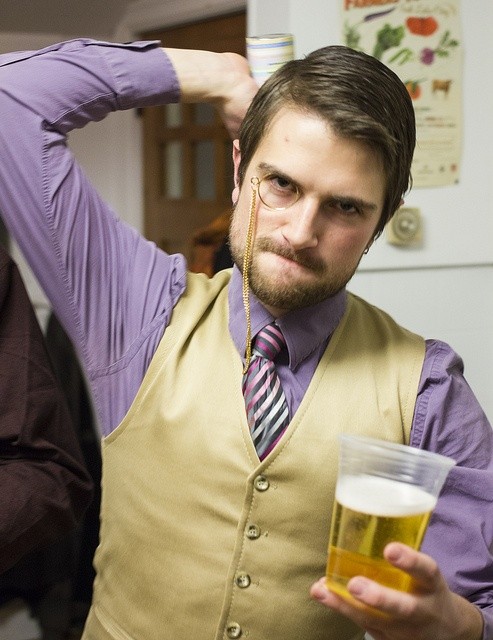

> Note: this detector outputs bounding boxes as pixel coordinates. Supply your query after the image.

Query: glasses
[244,170,300,378]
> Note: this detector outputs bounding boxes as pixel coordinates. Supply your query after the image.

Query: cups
[326,434,457,606]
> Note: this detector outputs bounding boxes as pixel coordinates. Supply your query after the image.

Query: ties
[244,324,291,462]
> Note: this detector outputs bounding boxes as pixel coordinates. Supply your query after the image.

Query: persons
[0,251,94,580]
[1,39,491,640]
